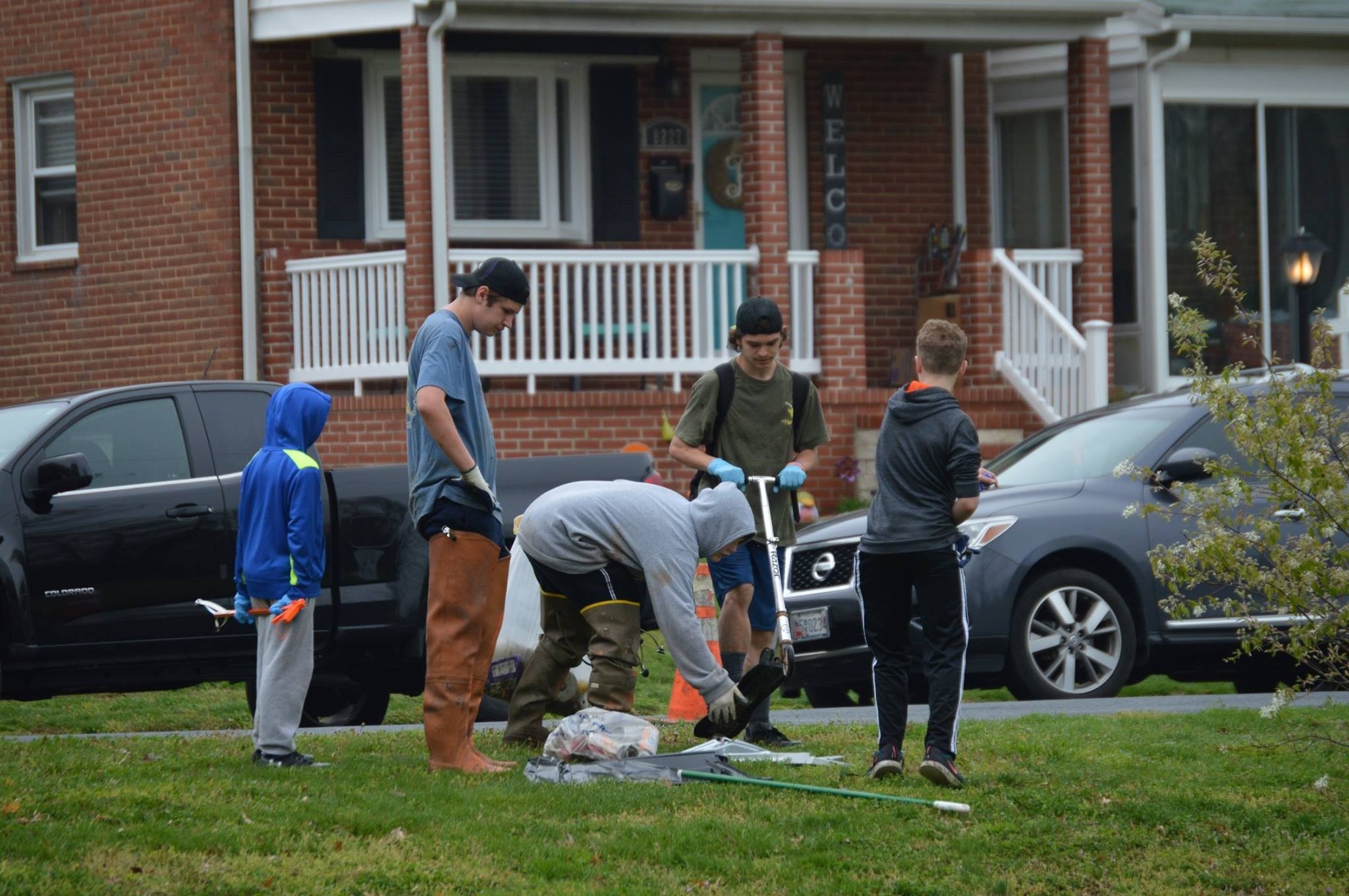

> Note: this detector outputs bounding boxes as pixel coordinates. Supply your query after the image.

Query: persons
[406,257,529,775]
[501,479,760,748]
[668,295,830,745]
[238,381,331,768]
[867,321,999,787]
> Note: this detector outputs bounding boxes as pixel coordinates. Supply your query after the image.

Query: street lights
[1276,233,1327,365]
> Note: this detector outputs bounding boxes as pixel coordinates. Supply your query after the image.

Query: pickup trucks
[1,380,664,699]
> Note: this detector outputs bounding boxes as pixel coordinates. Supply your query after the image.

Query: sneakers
[868,742,905,779]
[746,725,801,746]
[919,746,967,790]
[254,750,332,767]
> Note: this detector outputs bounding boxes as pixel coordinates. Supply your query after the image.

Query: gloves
[268,595,293,615]
[772,465,807,494]
[234,593,257,623]
[451,464,496,511]
[951,534,981,568]
[708,685,750,725]
[707,459,746,492]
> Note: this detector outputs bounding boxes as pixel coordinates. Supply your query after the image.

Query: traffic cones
[667,561,722,719]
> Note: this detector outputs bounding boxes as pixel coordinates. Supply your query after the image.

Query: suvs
[787,363,1349,706]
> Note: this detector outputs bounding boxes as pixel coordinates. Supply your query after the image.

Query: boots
[423,529,511,773]
[580,600,641,712]
[503,591,591,747]
[467,546,517,768]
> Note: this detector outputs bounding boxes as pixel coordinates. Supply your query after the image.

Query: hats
[451,258,531,305]
[736,296,783,333]
